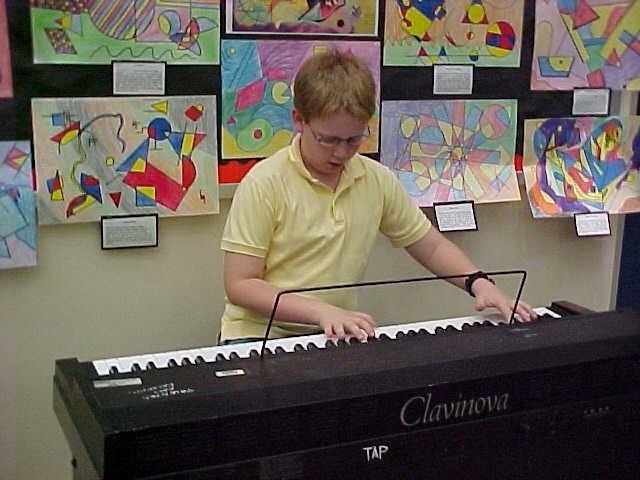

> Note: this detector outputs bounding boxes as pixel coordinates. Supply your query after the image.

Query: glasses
[307,123,371,146]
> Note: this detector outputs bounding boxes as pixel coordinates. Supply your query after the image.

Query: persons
[218,50,537,344]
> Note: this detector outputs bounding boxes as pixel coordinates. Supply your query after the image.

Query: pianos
[53,298,640,480]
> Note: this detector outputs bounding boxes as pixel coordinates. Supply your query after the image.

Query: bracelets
[465,271,496,298]
